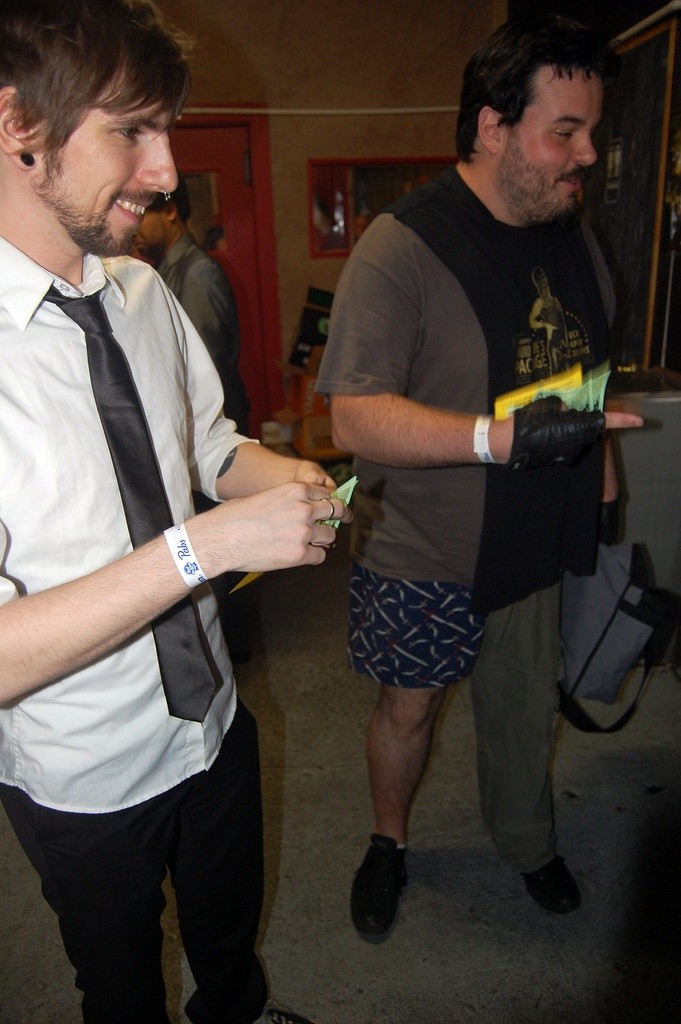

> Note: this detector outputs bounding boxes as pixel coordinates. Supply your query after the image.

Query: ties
[45,288,222,724]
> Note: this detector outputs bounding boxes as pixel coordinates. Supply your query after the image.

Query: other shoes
[251,996,314,1024]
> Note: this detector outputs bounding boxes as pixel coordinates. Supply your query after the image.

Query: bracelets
[163,523,209,589]
[473,415,496,464]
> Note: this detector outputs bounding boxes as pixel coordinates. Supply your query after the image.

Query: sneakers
[349,833,405,936]
[522,853,582,913]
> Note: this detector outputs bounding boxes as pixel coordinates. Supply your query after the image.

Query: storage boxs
[288,346,352,458]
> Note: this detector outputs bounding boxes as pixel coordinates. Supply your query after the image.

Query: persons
[132,172,256,664]
[0,0,354,1024]
[312,12,647,944]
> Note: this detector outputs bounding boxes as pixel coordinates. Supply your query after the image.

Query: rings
[321,498,334,519]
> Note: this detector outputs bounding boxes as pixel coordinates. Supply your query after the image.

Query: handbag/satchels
[562,539,678,706]
